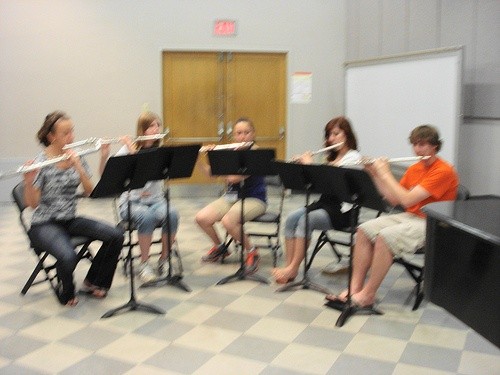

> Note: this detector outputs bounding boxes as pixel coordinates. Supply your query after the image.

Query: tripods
[138,144,202,293]
[326,165,384,327]
[208,147,277,288]
[276,160,330,296]
[90,149,164,321]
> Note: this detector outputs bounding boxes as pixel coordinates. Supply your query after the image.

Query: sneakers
[245,248,260,275]
[201,241,232,261]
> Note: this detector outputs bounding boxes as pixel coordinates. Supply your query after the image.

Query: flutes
[199,141,254,152]
[353,155,432,165]
[102,134,166,144]
[288,142,344,163]
[0,137,101,181]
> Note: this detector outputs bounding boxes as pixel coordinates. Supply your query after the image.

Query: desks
[419,199,500,349]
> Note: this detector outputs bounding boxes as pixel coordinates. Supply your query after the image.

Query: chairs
[220,175,286,268]
[391,184,469,310]
[113,194,183,276]
[306,208,381,272]
[12,179,95,305]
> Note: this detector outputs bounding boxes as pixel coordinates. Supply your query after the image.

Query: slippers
[351,296,374,310]
[79,285,107,298]
[57,286,78,306]
[325,293,347,303]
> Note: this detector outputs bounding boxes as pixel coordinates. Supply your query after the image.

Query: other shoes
[157,260,175,278]
[137,261,157,283]
[322,256,349,274]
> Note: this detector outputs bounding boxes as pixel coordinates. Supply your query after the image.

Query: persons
[195,118,268,274]
[272,117,364,284]
[325,125,457,310]
[99,113,179,283]
[21,111,125,307]
